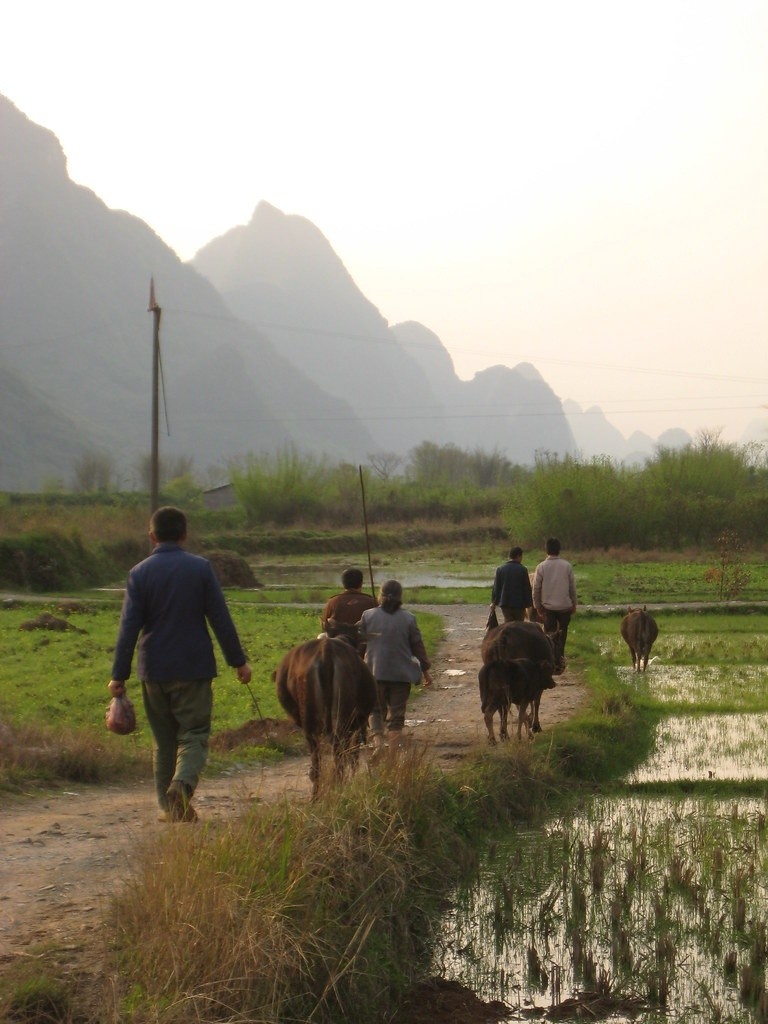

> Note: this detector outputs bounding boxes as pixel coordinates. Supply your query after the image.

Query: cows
[622,604,659,672]
[480,621,567,734]
[478,657,558,744]
[271,637,379,808]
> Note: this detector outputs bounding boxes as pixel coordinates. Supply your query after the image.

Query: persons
[107,506,251,823]
[533,538,577,665]
[324,569,379,655]
[488,546,533,621]
[359,578,432,759]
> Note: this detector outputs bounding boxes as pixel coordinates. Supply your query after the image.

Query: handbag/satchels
[106,685,136,734]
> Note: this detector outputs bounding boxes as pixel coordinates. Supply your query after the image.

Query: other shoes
[158,783,198,823]
[486,607,498,631]
